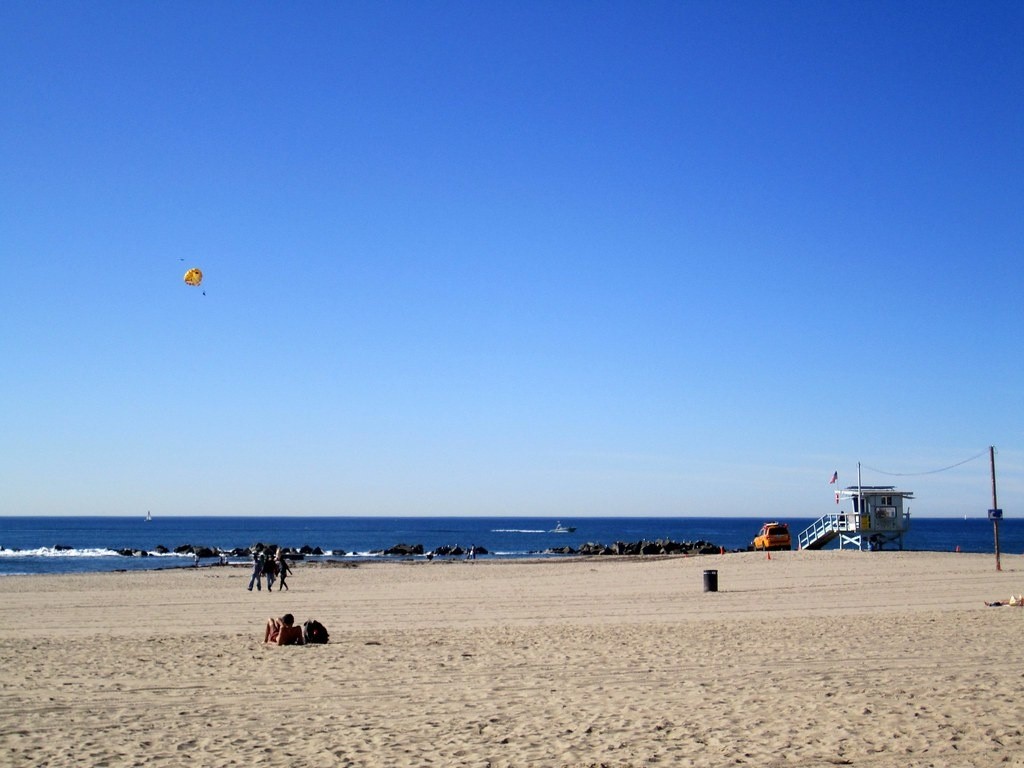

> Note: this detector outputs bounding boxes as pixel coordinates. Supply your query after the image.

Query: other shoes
[248,587,252,591]
[258,587,261,590]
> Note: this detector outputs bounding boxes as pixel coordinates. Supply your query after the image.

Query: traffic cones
[766,552,771,560]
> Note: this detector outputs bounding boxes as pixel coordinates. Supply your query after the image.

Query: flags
[830,471,837,483]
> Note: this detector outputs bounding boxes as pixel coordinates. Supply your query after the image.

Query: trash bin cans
[703,569,718,592]
[720,546,724,555]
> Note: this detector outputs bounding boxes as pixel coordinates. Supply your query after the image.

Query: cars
[753,523,792,551]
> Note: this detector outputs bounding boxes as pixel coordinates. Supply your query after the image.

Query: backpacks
[304,620,329,643]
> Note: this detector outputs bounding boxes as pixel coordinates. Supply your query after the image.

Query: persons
[225,555,228,564]
[467,544,476,559]
[248,552,292,591]
[261,614,303,646]
[219,557,223,564]
